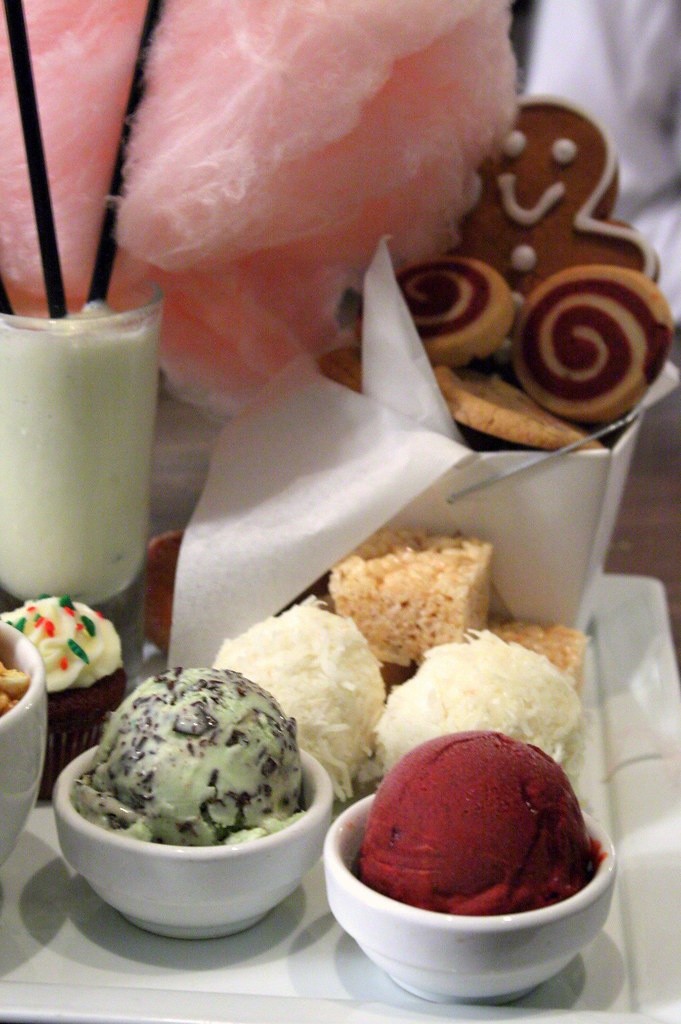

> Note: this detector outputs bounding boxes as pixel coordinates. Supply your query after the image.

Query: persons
[527,1,681,327]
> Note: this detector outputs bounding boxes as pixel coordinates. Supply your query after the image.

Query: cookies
[393,91,677,448]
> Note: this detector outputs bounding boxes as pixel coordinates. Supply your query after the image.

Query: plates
[0,571,681,1024]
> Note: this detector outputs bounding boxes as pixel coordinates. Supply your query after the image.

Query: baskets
[37,669,127,798]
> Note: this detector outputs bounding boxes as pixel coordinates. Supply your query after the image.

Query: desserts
[66,666,308,847]
[0,594,128,801]
[199,527,590,813]
[348,729,594,917]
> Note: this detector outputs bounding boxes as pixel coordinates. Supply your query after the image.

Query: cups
[1,276,164,692]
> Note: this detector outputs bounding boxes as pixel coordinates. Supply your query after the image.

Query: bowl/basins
[324,794,618,1007]
[0,621,49,870]
[35,669,131,808]
[51,745,335,939]
